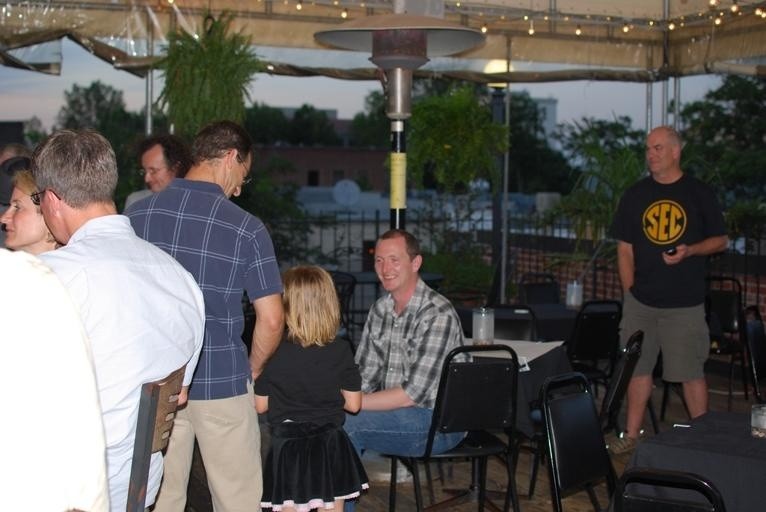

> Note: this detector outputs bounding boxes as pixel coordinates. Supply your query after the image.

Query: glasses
[28,188,60,206]
[227,149,255,187]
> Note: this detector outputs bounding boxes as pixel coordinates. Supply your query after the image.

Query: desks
[459,299,581,378]
[459,331,567,509]
[349,268,445,328]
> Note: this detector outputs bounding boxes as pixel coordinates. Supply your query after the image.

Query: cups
[565,279,583,308]
[471,307,495,348]
[751,404,766,439]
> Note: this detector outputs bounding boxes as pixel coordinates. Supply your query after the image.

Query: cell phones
[666,247,676,255]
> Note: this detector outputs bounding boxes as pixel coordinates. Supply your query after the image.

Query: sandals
[608,434,639,454]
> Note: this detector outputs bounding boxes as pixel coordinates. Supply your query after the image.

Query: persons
[1,170,64,255]
[0,154,38,175]
[34,126,207,511]
[608,127,729,453]
[1,141,38,162]
[1,242,110,510]
[123,133,186,216]
[119,122,286,512]
[254,267,364,512]
[343,231,472,511]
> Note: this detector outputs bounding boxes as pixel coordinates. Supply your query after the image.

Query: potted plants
[441,255,492,311]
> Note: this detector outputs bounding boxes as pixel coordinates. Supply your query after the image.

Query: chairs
[312,267,359,348]
[375,270,766,510]
[124,362,192,512]
